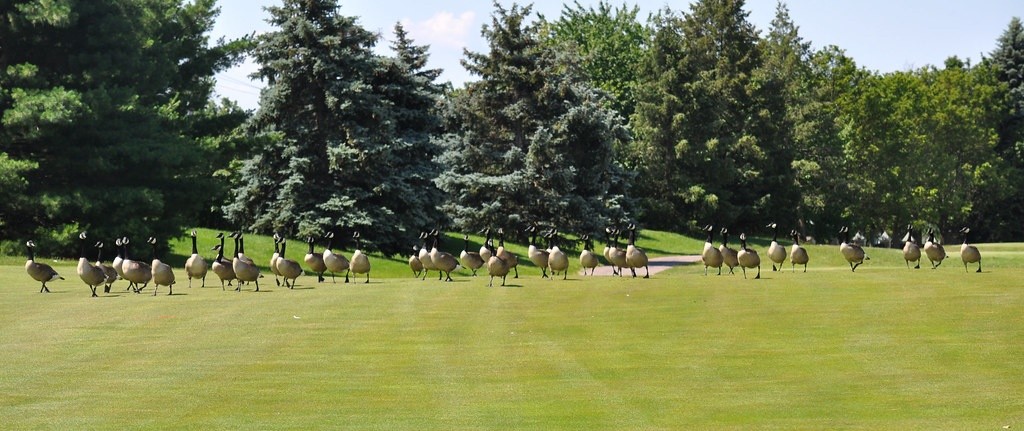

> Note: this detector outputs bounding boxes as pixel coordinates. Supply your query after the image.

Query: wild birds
[904,223,921,269]
[26,240,65,293]
[77,229,519,298]
[790,229,809,273]
[765,222,787,271]
[626,222,649,279]
[580,235,597,275]
[524,223,569,279]
[839,226,870,272]
[959,224,981,273]
[924,227,949,269]
[603,226,629,277]
[701,224,761,280]
